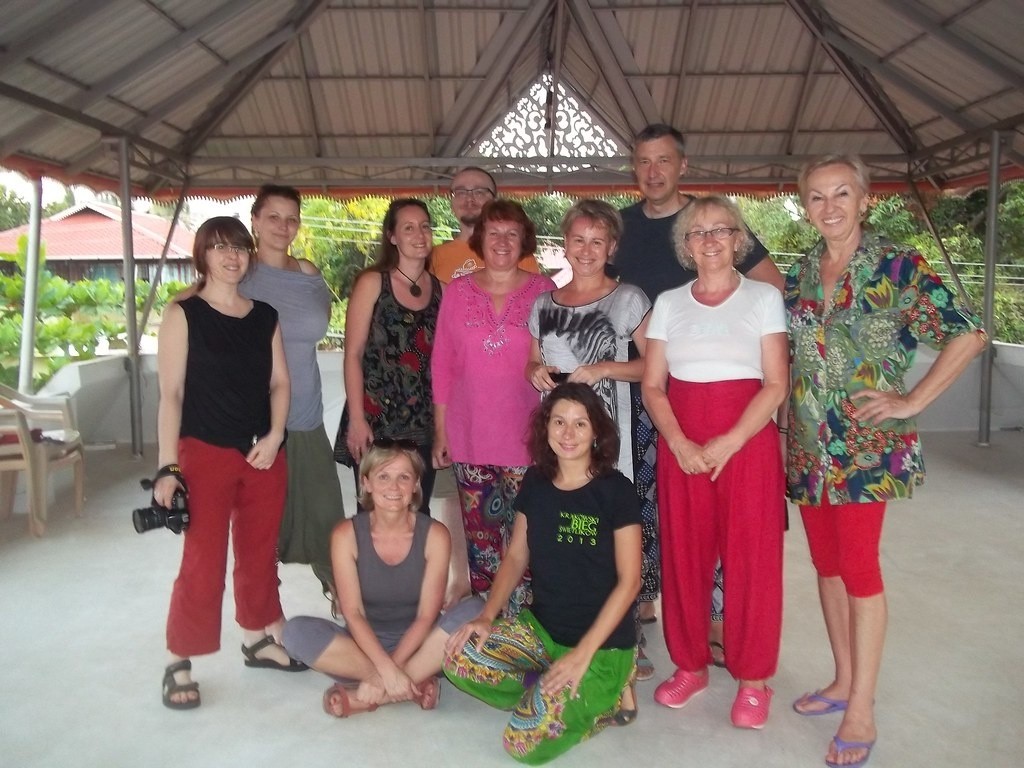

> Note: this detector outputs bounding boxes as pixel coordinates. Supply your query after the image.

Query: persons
[430,199,560,620]
[640,193,790,730]
[170,183,351,622]
[441,381,645,767]
[279,436,487,718]
[525,197,656,682]
[777,150,990,768]
[427,167,539,285]
[606,123,785,668]
[152,216,309,709]
[332,198,449,518]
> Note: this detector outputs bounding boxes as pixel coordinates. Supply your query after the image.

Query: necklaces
[396,267,426,297]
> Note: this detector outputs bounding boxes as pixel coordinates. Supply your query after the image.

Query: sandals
[242,636,309,673]
[609,681,636,725]
[413,676,442,710]
[162,660,201,710]
[323,681,378,716]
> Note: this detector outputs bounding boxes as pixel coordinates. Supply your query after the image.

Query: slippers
[634,658,654,681]
[709,641,727,671]
[638,603,657,624]
[825,734,877,768]
[793,689,875,716]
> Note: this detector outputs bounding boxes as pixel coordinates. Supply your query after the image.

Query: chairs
[0,383,86,541]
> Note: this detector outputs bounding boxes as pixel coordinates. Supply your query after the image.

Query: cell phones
[549,372,572,384]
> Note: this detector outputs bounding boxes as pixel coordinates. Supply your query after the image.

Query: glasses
[451,187,495,198]
[684,228,741,242]
[206,243,252,255]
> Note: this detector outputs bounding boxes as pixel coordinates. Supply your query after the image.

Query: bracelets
[778,427,788,434]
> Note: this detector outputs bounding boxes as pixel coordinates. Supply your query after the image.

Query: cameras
[132,491,190,534]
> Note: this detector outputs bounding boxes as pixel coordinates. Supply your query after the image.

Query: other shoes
[731,684,774,728]
[653,665,708,707]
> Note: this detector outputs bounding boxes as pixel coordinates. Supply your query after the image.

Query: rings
[355,448,360,450]
[690,470,695,473]
